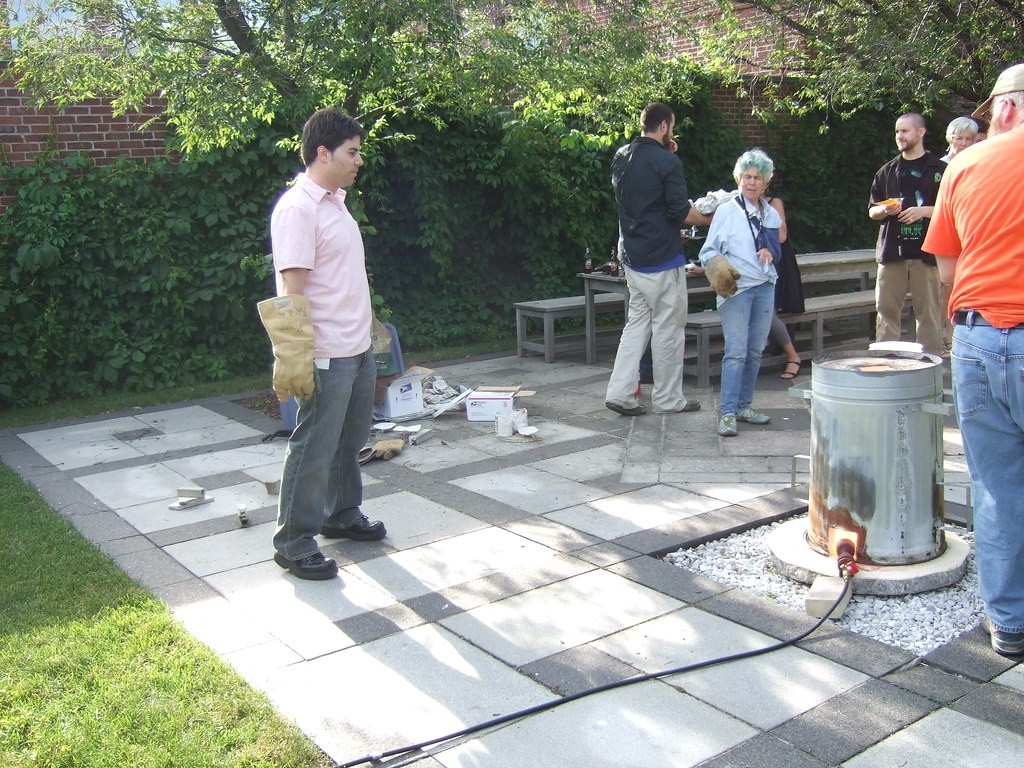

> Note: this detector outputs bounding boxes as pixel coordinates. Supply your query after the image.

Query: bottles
[611,245,619,276]
[584,245,592,274]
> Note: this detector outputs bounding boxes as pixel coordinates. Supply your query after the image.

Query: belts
[956,309,1024,329]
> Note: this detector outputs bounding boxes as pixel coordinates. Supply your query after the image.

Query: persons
[257,110,387,580]
[921,64,1024,657]
[698,149,771,436]
[605,103,714,417]
[612,143,654,384]
[969,117,990,145]
[867,114,948,357]
[940,116,978,165]
[688,195,805,379]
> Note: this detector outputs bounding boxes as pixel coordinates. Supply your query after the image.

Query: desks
[579,249,916,364]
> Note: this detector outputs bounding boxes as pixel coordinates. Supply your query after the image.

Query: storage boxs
[372,365,433,418]
[465,385,537,421]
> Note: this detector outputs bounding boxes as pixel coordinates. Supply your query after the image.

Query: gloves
[257,295,314,403]
[703,253,740,299]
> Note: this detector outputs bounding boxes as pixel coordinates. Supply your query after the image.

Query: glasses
[981,99,1004,126]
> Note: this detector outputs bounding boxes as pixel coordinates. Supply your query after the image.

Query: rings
[908,218,913,222]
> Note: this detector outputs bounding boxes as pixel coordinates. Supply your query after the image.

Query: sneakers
[982,612,1023,653]
[718,413,737,436]
[321,516,388,541]
[605,402,646,415]
[677,399,701,412]
[274,551,336,580]
[736,407,770,423]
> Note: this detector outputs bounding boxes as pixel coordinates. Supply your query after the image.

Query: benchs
[681,289,915,389]
[512,270,878,370]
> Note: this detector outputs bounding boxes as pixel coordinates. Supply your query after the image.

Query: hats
[971,63,1024,121]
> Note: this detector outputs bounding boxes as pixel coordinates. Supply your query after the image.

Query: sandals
[781,362,800,379]
